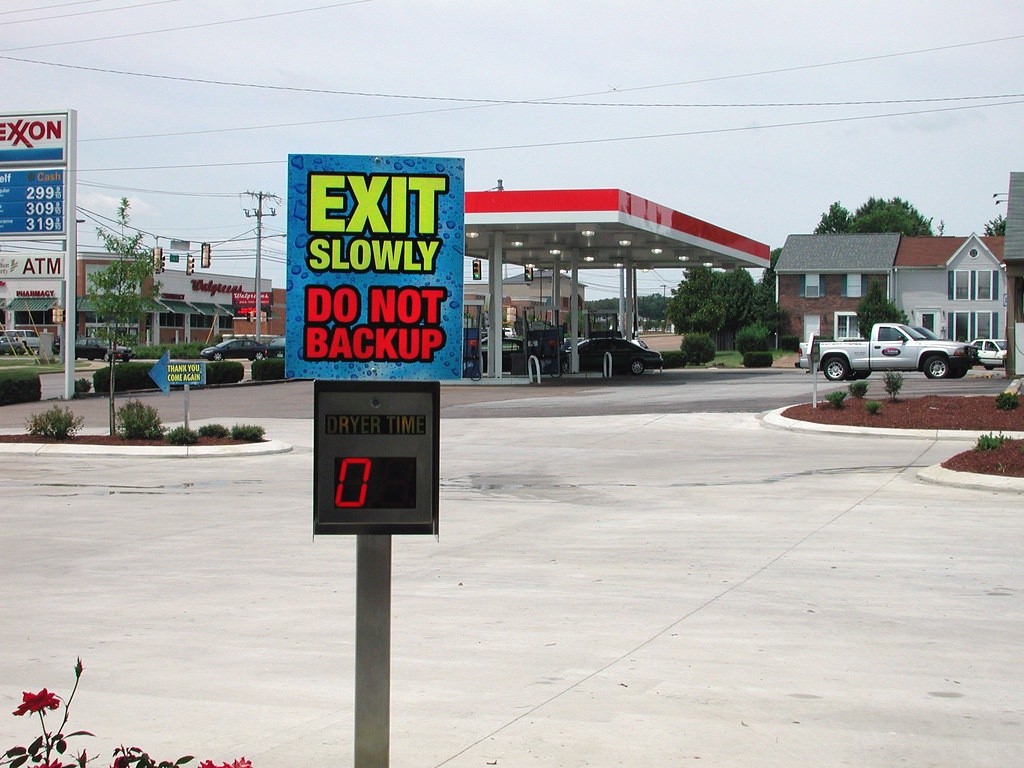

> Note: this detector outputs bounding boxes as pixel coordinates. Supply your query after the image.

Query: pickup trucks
[794,323,981,381]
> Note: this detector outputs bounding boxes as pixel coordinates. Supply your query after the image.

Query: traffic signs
[147,349,206,396]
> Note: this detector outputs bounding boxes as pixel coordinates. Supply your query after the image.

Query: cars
[268,338,285,358]
[969,338,1008,370]
[0,336,27,355]
[199,338,268,361]
[481,327,664,376]
[74,337,132,363]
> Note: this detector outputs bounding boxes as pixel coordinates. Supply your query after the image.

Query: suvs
[2,329,40,350]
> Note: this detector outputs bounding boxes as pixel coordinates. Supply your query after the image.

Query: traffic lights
[472,260,481,280]
[247,312,253,323]
[525,265,534,281]
[155,256,166,274]
[186,255,195,276]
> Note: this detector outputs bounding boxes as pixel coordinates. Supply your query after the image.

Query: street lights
[660,284,667,334]
[254,233,287,343]
